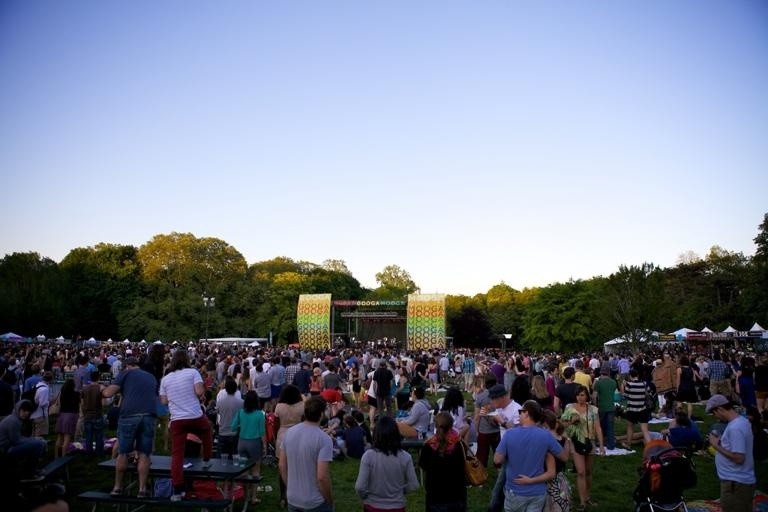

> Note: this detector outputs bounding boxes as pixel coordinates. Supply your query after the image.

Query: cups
[232,455,240,466]
[711,430,721,446]
[221,454,228,466]
[240,457,247,467]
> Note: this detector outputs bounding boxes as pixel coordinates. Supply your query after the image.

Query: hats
[705,393,730,414]
[313,367,322,377]
[488,384,508,399]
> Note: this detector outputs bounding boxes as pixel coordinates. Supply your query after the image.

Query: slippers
[576,503,586,511]
[586,498,598,507]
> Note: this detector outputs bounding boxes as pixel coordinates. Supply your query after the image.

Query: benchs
[78,492,231,512]
[135,472,264,483]
[42,454,73,480]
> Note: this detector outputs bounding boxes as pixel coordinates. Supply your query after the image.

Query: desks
[98,454,256,512]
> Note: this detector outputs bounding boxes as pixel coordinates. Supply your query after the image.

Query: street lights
[201,296,215,338]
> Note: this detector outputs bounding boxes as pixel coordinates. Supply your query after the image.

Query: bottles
[418,426,423,440]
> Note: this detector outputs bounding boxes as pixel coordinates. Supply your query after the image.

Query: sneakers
[201,461,214,468]
[109,486,123,495]
[137,490,152,498]
[170,494,182,501]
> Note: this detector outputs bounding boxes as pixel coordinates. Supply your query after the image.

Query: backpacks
[21,384,47,416]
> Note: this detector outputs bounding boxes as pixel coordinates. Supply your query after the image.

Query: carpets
[683,490,768,512]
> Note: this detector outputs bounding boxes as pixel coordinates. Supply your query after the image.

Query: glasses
[518,409,527,415]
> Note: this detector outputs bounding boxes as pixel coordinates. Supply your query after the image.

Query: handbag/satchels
[645,393,656,411]
[49,391,60,414]
[573,436,592,455]
[463,448,489,487]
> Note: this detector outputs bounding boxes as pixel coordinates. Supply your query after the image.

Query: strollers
[629,444,693,512]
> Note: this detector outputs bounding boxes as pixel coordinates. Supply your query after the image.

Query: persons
[0,335,767,511]
